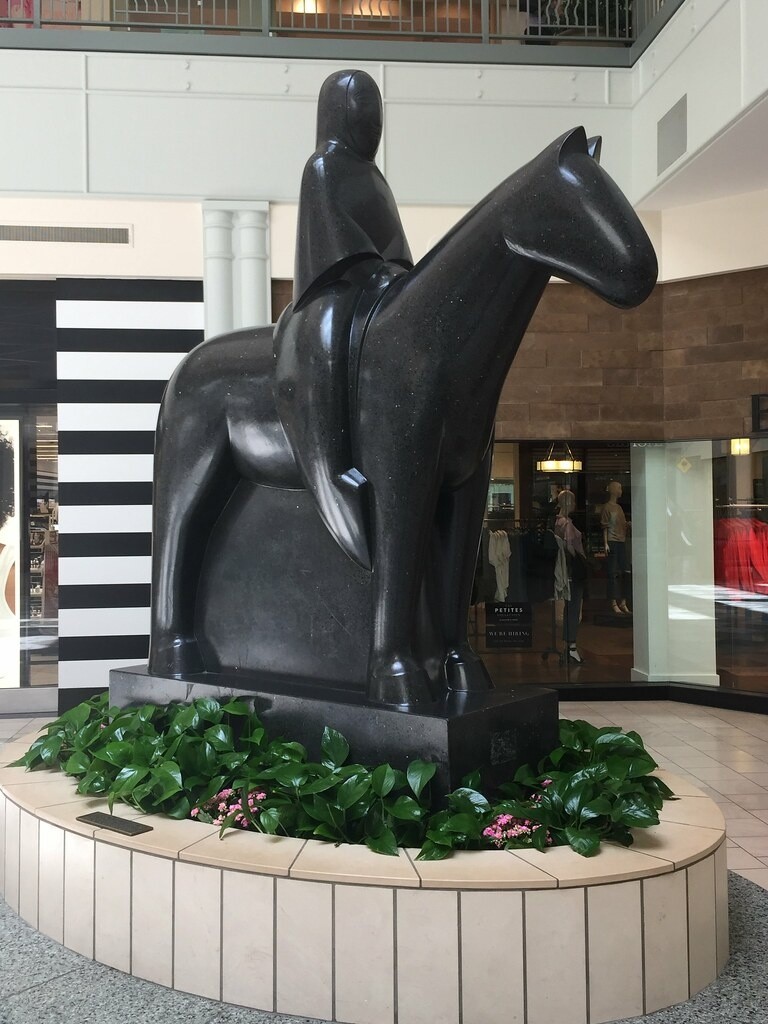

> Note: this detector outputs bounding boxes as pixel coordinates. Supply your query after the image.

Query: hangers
[718,502,765,528]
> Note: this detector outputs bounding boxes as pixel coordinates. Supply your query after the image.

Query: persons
[1,420,20,689]
[602,483,634,614]
[554,490,589,672]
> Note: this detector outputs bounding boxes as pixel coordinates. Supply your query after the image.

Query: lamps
[536,441,582,473]
[730,439,750,456]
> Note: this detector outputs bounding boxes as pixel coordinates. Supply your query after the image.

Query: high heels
[569,648,584,666]
[609,604,625,615]
[619,604,633,615]
